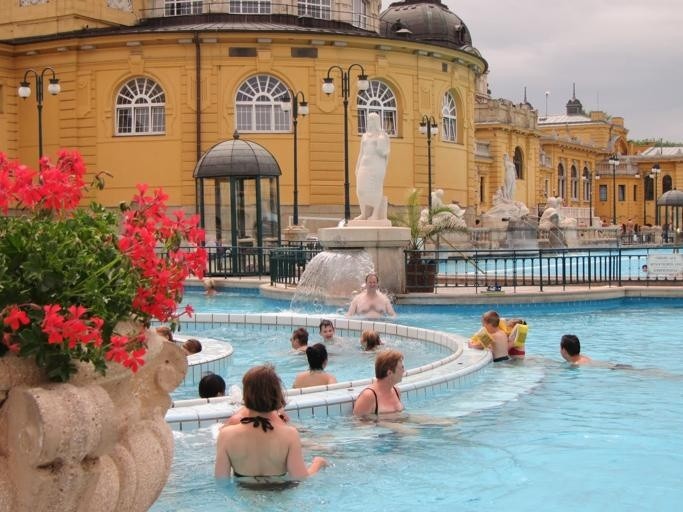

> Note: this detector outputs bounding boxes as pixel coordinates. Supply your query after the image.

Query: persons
[199,371,226,398]
[468,310,683,382]
[503,153,515,201]
[180,339,201,356]
[291,319,383,389]
[157,327,174,342]
[352,351,458,436]
[342,274,396,320]
[215,364,329,500]
[354,112,390,220]
[219,404,290,426]
[431,189,465,217]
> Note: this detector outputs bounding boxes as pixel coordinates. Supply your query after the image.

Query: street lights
[634,170,654,225]
[17,67,59,186]
[581,169,599,228]
[418,115,439,272]
[279,87,308,245]
[321,63,370,227]
[608,151,620,223]
[651,163,660,225]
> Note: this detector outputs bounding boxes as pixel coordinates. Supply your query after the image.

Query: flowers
[0,147,214,384]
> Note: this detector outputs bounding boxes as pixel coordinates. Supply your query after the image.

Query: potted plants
[387,189,472,293]
